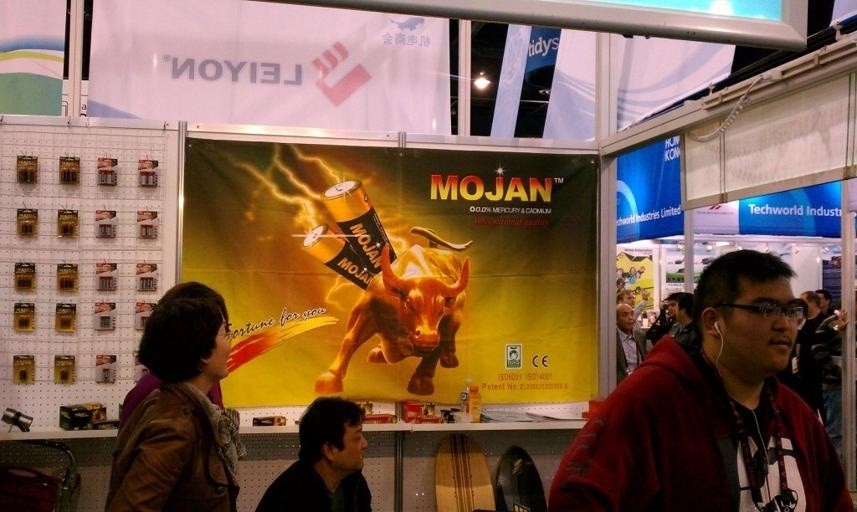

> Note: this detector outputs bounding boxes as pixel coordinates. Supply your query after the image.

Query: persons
[612,283,848,443]
[115,281,225,428]
[94,298,240,510]
[254,395,373,510]
[547,249,854,510]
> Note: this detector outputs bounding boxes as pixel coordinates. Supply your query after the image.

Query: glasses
[714,302,804,321]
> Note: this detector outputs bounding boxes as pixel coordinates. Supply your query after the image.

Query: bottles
[460,378,483,423]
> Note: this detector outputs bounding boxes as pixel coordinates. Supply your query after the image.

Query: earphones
[714,321,721,334]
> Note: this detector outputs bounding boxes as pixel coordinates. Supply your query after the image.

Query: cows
[314,243,469,397]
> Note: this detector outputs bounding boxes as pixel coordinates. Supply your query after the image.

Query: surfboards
[435,432,496,512]
[495,447,548,512]
